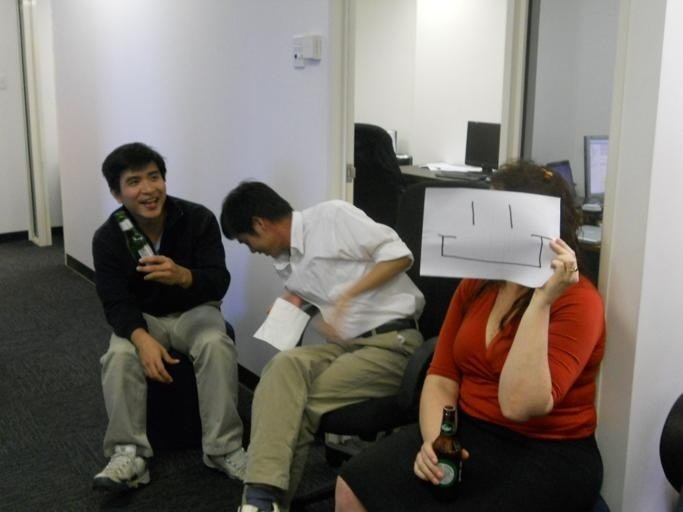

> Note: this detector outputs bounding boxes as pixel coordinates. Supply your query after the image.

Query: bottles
[428,404,465,512]
[114,209,158,281]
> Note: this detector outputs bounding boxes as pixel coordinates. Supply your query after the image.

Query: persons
[89,140,249,493]
[219,177,428,512]
[332,157,603,512]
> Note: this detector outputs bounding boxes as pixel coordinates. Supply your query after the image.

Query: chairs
[306,319,438,509]
[354,121,439,242]
[146,318,238,399]
[658,391,682,497]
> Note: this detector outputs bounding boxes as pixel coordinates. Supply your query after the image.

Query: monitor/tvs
[584,135,608,209]
[547,159,581,209]
[465,121,500,175]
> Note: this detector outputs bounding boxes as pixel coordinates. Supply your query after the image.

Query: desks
[396,162,603,263]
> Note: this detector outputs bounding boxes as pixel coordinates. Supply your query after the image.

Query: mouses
[485,176,492,183]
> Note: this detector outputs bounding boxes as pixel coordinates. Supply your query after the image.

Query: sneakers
[203,446,248,483]
[91,444,151,490]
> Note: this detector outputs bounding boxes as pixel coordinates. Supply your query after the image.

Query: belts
[355,319,416,338]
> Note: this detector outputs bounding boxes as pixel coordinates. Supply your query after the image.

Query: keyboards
[436,170,481,181]
[576,224,601,245]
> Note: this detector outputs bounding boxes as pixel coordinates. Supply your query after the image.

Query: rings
[567,266,578,274]
